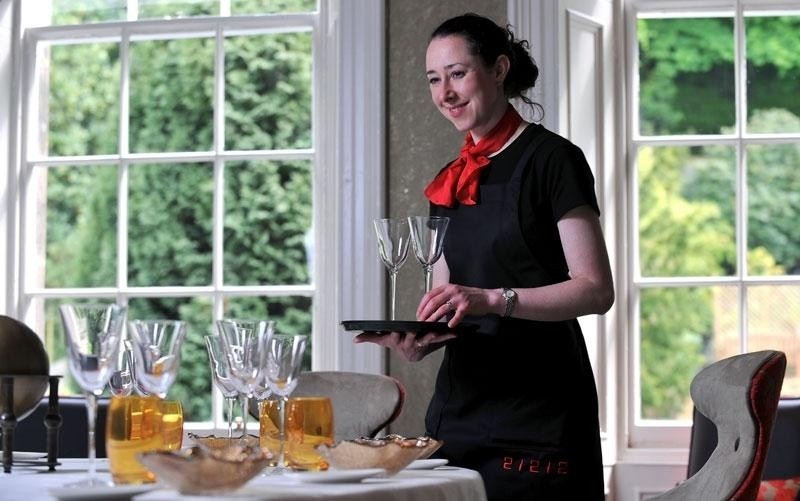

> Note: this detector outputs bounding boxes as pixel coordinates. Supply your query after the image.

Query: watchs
[500,287,518,320]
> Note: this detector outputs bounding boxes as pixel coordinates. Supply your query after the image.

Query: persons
[352,12,615,501]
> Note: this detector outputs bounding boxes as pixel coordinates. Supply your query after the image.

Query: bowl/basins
[316,433,447,479]
[136,448,273,494]
[188,432,261,463]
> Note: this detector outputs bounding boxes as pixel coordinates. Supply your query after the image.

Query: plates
[302,468,384,483]
[407,459,449,470]
[50,486,152,501]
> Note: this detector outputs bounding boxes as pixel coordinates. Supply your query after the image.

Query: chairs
[238,371,406,438]
[643,349,787,501]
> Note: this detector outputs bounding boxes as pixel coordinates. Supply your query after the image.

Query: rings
[448,299,458,312]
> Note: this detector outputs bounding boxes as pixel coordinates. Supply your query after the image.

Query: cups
[109,340,135,397]
[254,400,286,467]
[285,397,334,473]
[106,398,185,484]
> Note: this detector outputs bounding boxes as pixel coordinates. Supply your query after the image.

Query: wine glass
[372,215,450,320]
[203,320,308,476]
[124,320,189,483]
[59,303,130,485]
